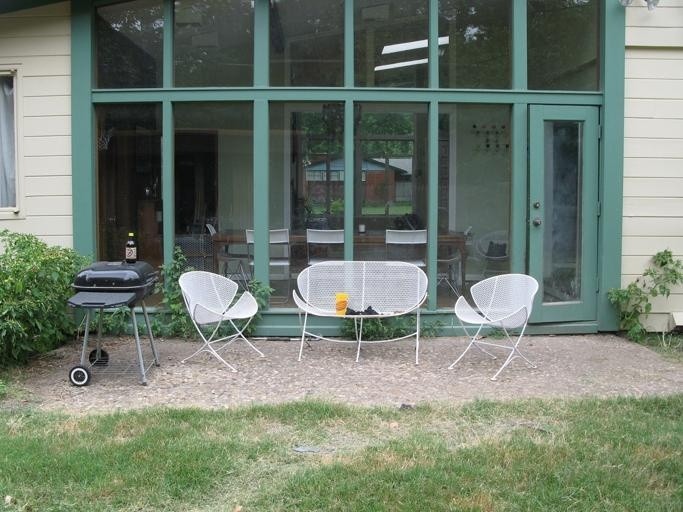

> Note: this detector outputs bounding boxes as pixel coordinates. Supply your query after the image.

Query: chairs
[476,230,509,280]
[449,273,539,381]
[178,270,265,373]
[205,224,474,305]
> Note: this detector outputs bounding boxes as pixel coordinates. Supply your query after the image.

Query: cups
[336,293,349,315]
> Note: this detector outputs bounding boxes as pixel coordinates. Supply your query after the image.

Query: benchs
[292,260,429,365]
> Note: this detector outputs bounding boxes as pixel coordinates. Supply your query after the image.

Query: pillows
[485,240,506,257]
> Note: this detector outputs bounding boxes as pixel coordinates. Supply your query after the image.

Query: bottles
[125,232,137,264]
